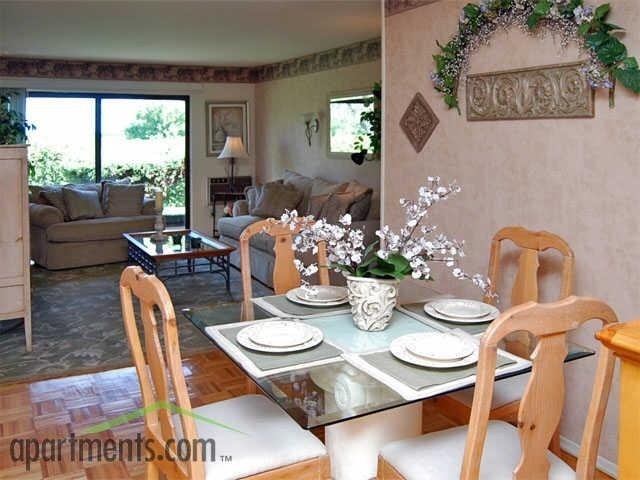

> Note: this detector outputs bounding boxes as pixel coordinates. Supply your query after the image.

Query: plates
[248,321,312,346]
[296,286,350,301]
[405,337,474,358]
[285,286,348,305]
[390,333,479,366]
[431,299,492,317]
[236,323,323,352]
[424,303,500,322]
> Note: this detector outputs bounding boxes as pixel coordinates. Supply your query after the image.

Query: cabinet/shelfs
[211,192,245,240]
[0,144,32,352]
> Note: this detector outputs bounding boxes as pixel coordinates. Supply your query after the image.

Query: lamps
[217,136,251,194]
[301,112,319,147]
[351,149,367,165]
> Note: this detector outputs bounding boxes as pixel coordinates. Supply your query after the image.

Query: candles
[154,191,163,208]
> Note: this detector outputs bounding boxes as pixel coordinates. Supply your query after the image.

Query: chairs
[436,226,574,459]
[119,265,331,480]
[240,218,330,396]
[376,294,618,480]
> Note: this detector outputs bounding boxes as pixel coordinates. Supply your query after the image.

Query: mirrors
[326,88,377,160]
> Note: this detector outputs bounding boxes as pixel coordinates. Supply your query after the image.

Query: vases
[345,276,400,333]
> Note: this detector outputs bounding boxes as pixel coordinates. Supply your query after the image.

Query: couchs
[217,171,379,289]
[29,180,155,270]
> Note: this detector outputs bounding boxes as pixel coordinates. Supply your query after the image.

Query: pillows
[102,183,145,217]
[64,184,100,196]
[251,183,304,218]
[244,185,261,213]
[307,176,348,198]
[280,169,313,195]
[317,192,361,224]
[29,185,57,204]
[40,190,70,222]
[345,179,373,222]
[61,187,104,219]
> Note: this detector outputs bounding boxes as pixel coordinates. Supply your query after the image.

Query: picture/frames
[205,101,250,158]
